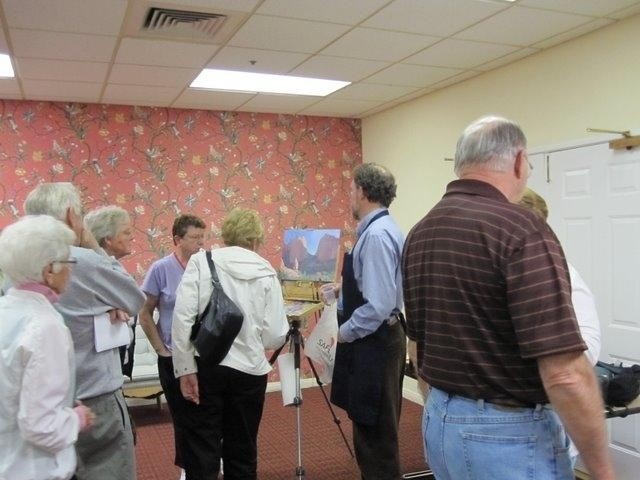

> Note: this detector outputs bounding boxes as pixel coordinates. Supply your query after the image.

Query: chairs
[122,309,164,410]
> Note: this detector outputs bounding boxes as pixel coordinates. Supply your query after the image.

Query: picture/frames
[278,228,341,283]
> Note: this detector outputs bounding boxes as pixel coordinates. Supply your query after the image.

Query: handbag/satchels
[185,247,245,362]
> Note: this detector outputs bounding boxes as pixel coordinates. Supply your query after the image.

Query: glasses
[51,256,79,268]
[522,152,535,177]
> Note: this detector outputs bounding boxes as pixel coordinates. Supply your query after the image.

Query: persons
[400,115,615,480]
[82,205,138,383]
[138,214,224,480]
[519,187,603,470]
[0,214,97,479]
[0,181,147,480]
[330,160,405,480]
[171,208,289,480]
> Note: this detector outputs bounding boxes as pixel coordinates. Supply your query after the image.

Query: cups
[321,285,335,305]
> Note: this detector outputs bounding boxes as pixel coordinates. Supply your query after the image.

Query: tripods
[262,317,354,480]
